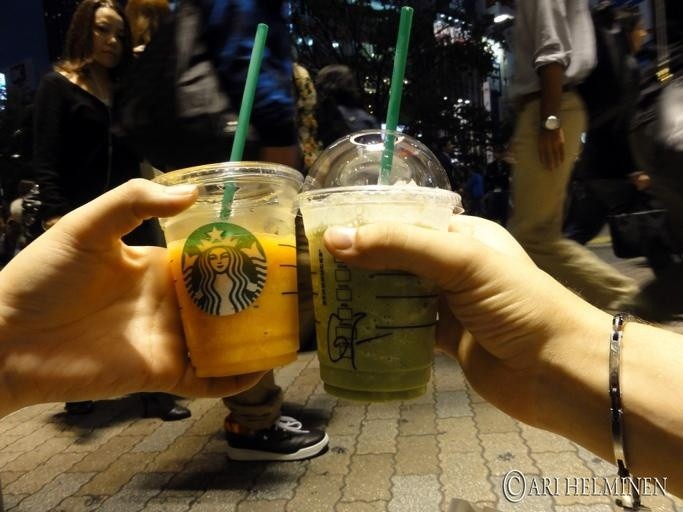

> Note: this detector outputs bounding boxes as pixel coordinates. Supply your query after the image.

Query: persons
[118,2,332,461]
[0,176,309,408]
[33,1,192,424]
[1,1,681,325]
[322,208,683,511]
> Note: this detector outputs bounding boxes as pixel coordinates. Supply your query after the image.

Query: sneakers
[224,413,330,461]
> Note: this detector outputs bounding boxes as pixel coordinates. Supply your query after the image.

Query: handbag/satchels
[629,54,683,183]
[608,208,683,259]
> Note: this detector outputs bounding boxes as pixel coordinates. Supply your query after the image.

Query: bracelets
[606,307,646,510]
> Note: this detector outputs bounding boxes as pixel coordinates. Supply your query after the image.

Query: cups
[293,184,466,403]
[144,160,306,380]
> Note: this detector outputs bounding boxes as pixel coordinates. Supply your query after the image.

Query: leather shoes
[66,401,92,414]
[144,396,191,419]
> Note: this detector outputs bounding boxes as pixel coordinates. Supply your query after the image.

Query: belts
[523,82,575,102]
